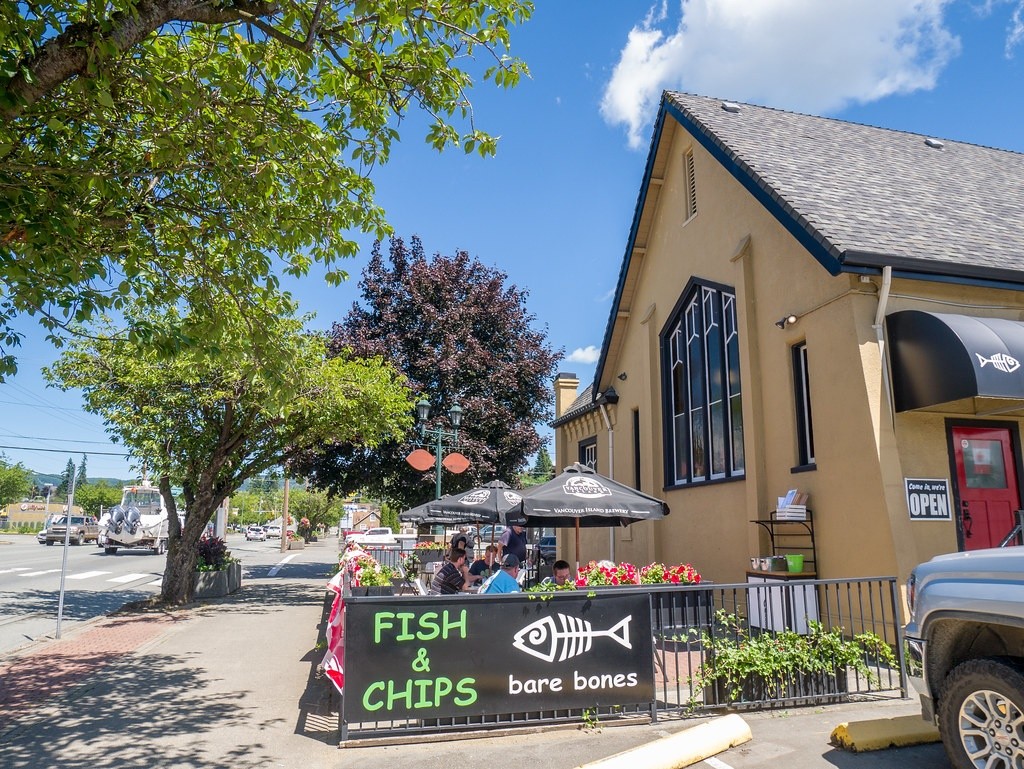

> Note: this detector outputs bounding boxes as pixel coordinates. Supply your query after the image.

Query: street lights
[407,397,471,536]
[43,483,54,514]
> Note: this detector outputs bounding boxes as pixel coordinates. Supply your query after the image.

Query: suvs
[903,535,1024,769]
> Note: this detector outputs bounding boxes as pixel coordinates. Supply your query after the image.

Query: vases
[416,550,445,561]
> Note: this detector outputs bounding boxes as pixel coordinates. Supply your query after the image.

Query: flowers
[415,541,451,550]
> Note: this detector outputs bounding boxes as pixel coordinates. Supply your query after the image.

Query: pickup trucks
[46,516,98,546]
[345,527,417,549]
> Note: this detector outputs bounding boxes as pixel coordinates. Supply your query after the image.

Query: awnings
[885,309,1024,418]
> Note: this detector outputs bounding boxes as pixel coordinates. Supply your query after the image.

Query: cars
[227,522,270,534]
[37,514,64,544]
[286,530,295,536]
[246,526,267,541]
[534,536,556,562]
[267,525,281,539]
[342,525,477,544]
[469,524,506,545]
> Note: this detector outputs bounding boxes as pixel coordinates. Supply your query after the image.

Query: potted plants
[681,607,903,714]
[182,527,241,598]
[348,561,394,597]
[287,534,305,550]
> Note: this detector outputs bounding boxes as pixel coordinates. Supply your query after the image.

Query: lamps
[618,373,627,380]
[775,314,797,330]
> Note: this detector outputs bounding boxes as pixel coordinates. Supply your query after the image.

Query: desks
[462,586,479,594]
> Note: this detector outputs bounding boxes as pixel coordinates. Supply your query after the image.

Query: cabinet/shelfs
[745,508,818,637]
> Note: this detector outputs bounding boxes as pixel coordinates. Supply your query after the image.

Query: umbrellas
[505,461,670,576]
[398,478,525,559]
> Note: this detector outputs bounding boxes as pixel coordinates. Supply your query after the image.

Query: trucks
[104,486,186,554]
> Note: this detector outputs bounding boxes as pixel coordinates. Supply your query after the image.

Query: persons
[468,545,501,588]
[476,554,521,595]
[450,526,476,569]
[497,525,528,569]
[539,560,575,586]
[430,548,471,595]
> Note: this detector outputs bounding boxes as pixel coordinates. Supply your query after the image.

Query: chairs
[420,562,435,588]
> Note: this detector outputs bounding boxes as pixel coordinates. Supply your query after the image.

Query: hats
[499,553,523,569]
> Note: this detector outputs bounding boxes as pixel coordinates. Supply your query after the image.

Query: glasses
[557,573,570,579]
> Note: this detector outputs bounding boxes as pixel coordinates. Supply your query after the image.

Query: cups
[785,554,804,572]
[751,557,767,571]
